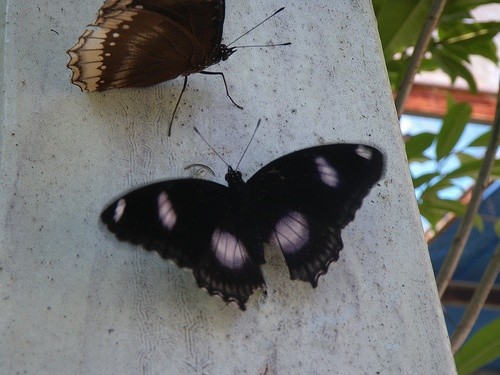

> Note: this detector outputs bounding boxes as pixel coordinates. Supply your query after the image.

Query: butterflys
[99,118,388,313]
[67,0,292,137]
